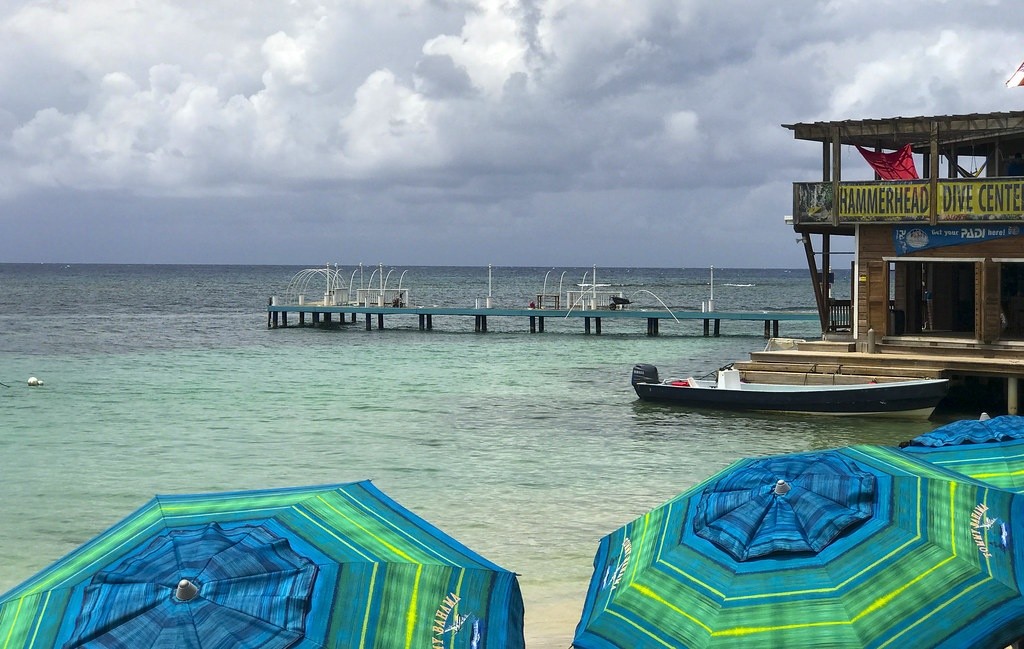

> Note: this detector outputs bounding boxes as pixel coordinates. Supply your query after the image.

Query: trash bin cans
[890,309,905,336]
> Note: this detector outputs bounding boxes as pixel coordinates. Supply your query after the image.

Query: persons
[1008,153,1024,176]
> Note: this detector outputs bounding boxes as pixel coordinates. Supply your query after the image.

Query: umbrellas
[898,412,1024,495]
[572,441,1024,649]
[0,479,525,649]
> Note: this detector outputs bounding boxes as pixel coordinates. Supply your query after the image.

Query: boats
[577,282,612,287]
[724,283,757,288]
[631,363,950,413]
